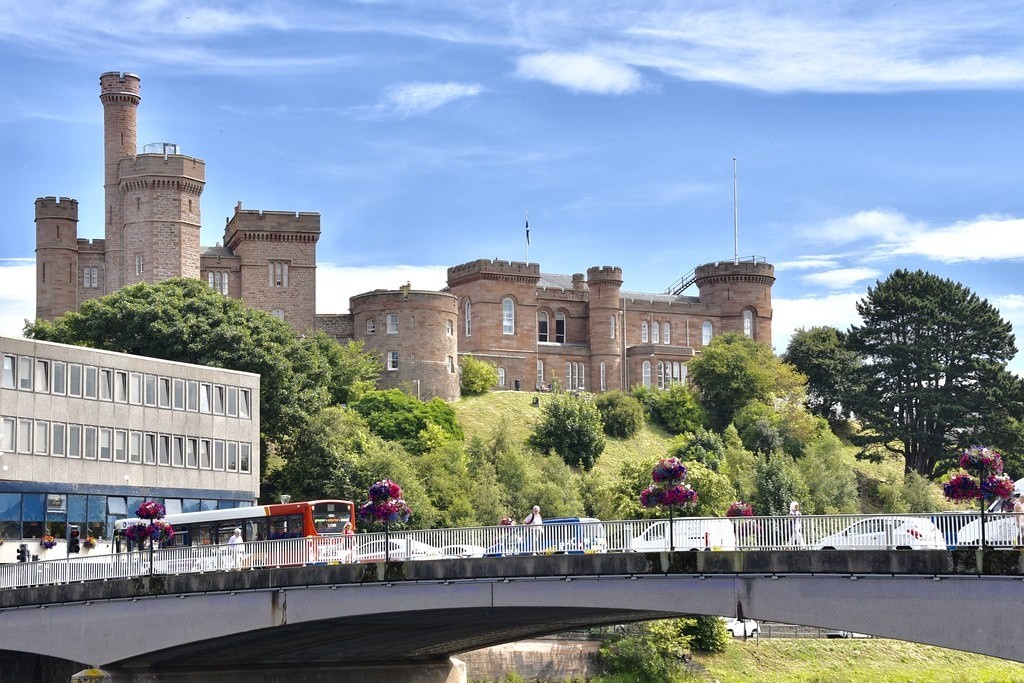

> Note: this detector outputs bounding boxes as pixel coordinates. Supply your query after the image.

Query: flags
[526,216,531,244]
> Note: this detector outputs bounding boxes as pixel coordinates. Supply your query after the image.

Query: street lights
[280,494,290,532]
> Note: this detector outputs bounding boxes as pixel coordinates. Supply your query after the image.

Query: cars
[813,517,948,550]
[354,539,486,560]
[824,629,875,639]
[717,617,761,638]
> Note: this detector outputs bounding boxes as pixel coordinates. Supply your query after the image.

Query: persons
[228,527,243,572]
[786,502,806,550]
[341,522,356,564]
[522,505,543,556]
[1014,495,1024,546]
[270,523,305,539]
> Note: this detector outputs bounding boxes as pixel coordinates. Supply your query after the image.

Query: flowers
[84,537,98,549]
[40,536,57,548]
[124,500,174,544]
[726,500,761,535]
[359,479,412,523]
[942,444,1014,503]
[639,456,697,507]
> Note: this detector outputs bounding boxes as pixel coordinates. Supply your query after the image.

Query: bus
[110,498,356,580]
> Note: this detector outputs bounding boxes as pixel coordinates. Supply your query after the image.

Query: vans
[957,478,1024,550]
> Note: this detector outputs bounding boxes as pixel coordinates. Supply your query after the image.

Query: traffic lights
[16,543,27,562]
[67,524,81,554]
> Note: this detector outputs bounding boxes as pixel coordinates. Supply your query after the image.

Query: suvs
[482,515,607,558]
[622,519,736,552]
